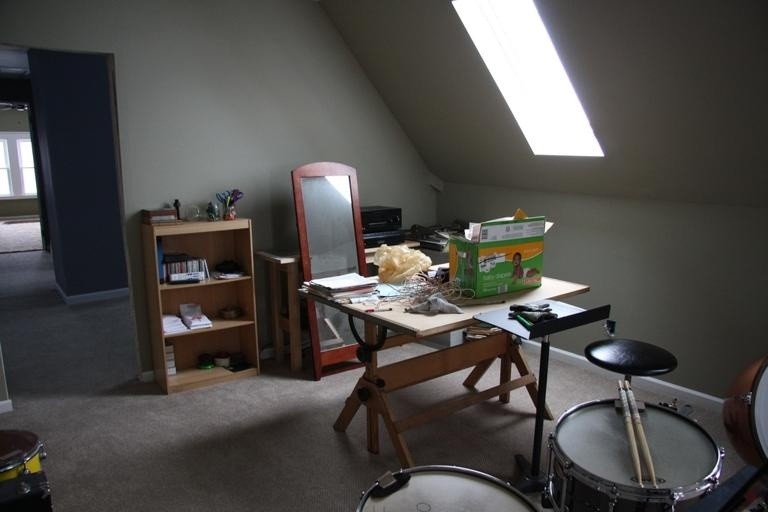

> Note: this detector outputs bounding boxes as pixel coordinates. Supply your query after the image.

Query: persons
[509,252,524,283]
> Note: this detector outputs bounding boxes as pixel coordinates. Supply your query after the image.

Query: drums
[356,464,544,512]
[723,354,768,470]
[544,396,725,512]
[0,430,46,482]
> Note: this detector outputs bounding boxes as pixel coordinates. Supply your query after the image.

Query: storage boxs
[436,209,554,298]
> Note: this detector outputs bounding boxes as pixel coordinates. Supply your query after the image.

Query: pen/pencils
[365,308,392,313]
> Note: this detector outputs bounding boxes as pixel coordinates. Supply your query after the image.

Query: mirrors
[291,161,372,380]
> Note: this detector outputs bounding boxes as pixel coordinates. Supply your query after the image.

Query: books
[156,235,246,339]
[305,272,379,301]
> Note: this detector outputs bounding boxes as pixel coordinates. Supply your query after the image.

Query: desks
[297,271,591,465]
[255,240,421,373]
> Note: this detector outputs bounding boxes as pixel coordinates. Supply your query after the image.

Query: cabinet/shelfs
[140,217,260,395]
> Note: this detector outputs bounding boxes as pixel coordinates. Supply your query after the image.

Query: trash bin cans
[0,429,54,511]
